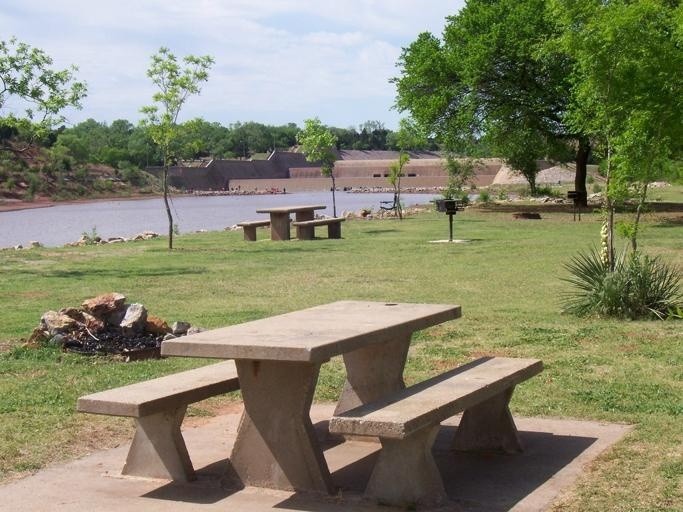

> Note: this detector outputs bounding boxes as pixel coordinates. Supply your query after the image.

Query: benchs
[292,217,346,240]
[76,359,241,483]
[237,218,292,241]
[328,356,544,507]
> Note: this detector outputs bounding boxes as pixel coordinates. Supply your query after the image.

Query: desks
[256,205,326,240]
[160,300,461,497]
